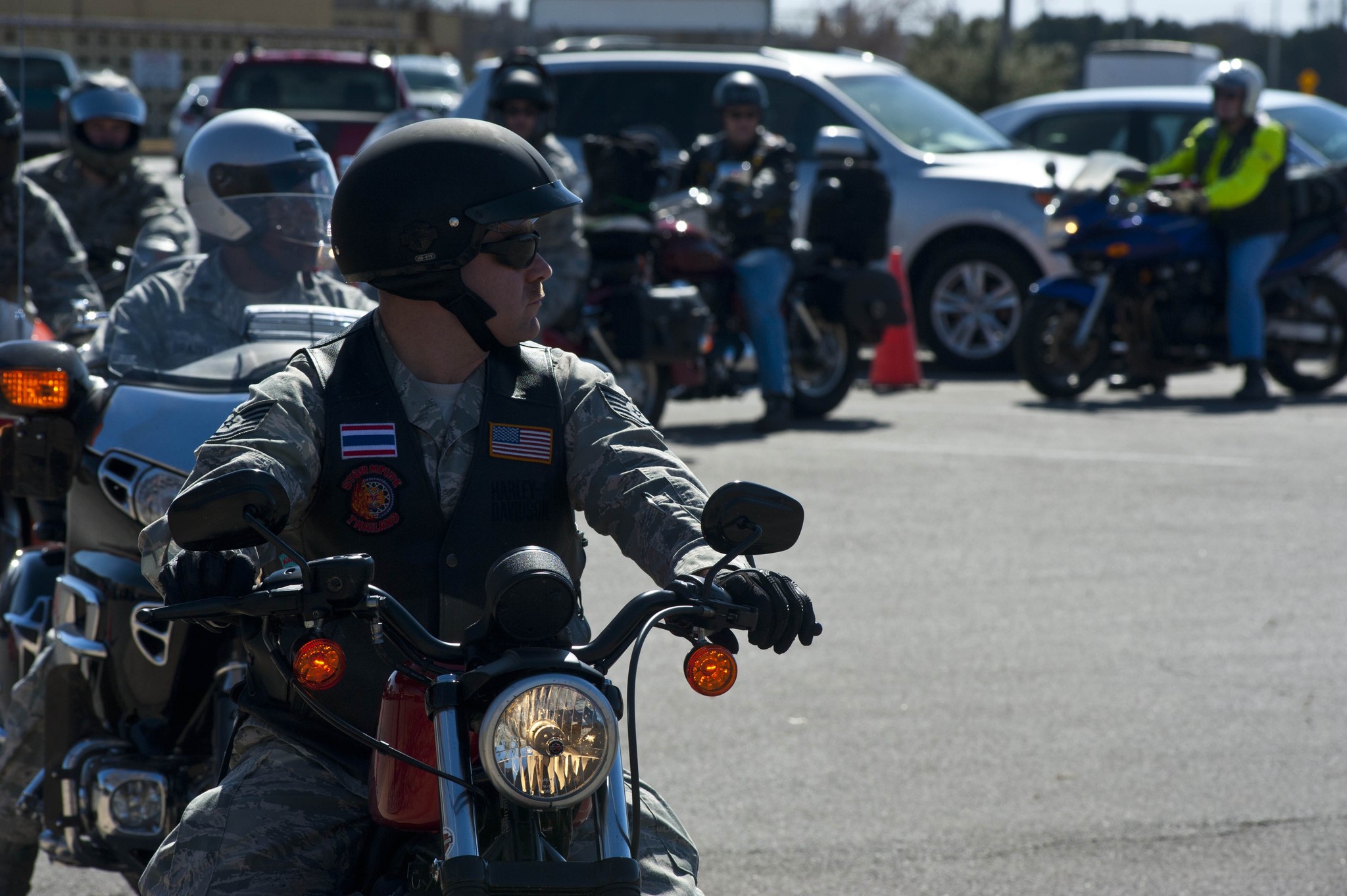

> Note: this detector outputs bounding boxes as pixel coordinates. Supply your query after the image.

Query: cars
[170,75,223,171]
[974,86,1347,339]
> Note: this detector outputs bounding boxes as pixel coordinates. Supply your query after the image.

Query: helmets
[66,70,148,174]
[0,78,25,140]
[488,53,559,115]
[714,71,770,110]
[332,116,583,283]
[181,108,326,242]
[1204,57,1264,119]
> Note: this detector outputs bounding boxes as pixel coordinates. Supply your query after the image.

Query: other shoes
[751,396,791,434]
[1107,371,1167,390]
[1235,375,1267,404]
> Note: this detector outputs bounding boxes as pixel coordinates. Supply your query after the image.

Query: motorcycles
[1010,149,1347,401]
[580,188,910,427]
[0,293,826,895]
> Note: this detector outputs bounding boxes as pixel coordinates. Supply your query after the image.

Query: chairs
[339,80,382,112]
[242,75,283,109]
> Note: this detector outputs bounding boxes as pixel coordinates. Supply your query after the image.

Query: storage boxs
[1278,156,1347,222]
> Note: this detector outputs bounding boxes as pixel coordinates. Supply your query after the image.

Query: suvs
[196,46,409,188]
[382,50,471,122]
[1,49,86,159]
[454,32,1130,382]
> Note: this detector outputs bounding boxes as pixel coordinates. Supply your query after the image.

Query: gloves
[705,570,823,655]
[158,547,256,633]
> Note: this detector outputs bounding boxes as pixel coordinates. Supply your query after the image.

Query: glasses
[480,230,544,268]
[1214,88,1236,101]
[505,107,534,116]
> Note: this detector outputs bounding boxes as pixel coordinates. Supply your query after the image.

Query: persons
[0,80,111,350]
[138,117,816,896]
[18,68,189,302]
[472,64,621,378]
[2,106,381,896]
[680,68,799,437]
[1114,56,1292,411]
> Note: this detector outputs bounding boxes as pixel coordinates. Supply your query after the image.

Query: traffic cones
[870,243,926,394]
